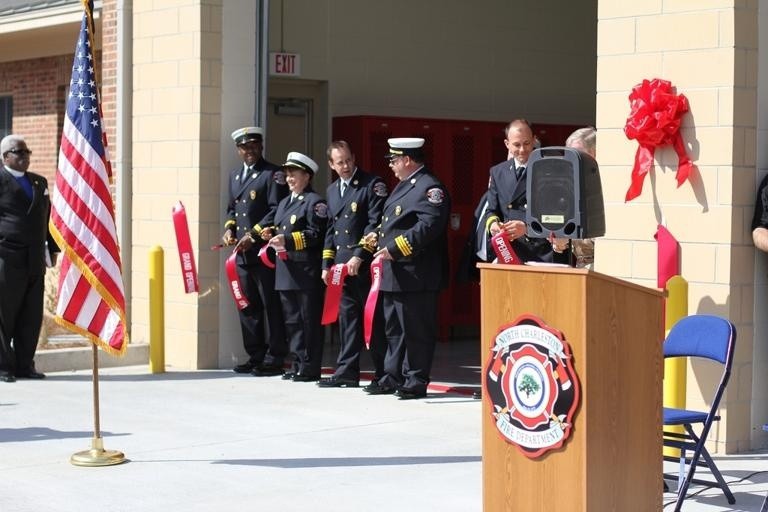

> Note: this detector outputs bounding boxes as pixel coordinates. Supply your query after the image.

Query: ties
[516,167,524,179]
[245,166,251,179]
[340,182,348,197]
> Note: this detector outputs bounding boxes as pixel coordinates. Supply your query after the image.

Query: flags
[50,1,129,359]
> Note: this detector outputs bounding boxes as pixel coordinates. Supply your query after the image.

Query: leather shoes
[395,388,427,398]
[317,376,359,387]
[293,372,321,381]
[16,371,45,379]
[364,382,396,394]
[283,369,296,379]
[473,390,482,399]
[233,360,258,374]
[251,363,285,376]
[0,371,17,381]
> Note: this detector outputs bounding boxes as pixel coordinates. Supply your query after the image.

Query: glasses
[390,157,402,164]
[4,149,31,154]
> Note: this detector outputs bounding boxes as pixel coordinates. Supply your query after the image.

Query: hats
[227,127,264,145]
[282,151,319,174]
[385,137,425,158]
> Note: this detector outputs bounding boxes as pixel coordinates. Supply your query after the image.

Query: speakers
[525,146,606,238]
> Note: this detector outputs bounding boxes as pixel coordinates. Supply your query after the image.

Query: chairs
[662,314,737,512]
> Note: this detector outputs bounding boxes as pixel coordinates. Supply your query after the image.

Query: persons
[318,141,386,387]
[0,134,60,383]
[347,137,448,400]
[549,126,598,265]
[484,119,542,265]
[261,153,327,381]
[222,127,287,377]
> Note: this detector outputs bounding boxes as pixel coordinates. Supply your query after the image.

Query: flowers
[622,76,692,205]
